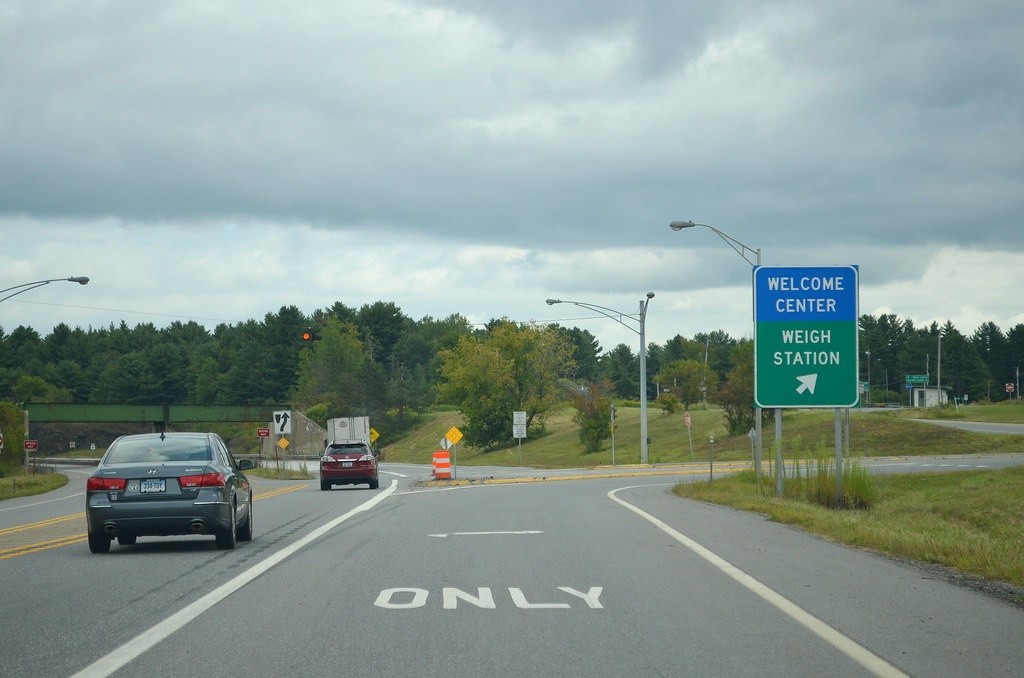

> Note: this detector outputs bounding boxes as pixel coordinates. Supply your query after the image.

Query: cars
[85,431,254,553]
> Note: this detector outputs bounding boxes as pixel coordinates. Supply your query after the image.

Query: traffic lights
[302,327,322,343]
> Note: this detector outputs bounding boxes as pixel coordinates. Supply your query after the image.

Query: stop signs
[1006,384,1014,391]
[684,411,691,428]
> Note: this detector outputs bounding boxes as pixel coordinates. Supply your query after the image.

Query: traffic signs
[754,320,860,407]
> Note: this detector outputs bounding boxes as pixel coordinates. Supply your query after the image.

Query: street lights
[938,335,945,408]
[865,351,872,408]
[1,277,89,304]
[669,221,763,482]
[546,292,655,462]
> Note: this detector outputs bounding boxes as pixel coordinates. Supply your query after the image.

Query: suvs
[318,438,380,491]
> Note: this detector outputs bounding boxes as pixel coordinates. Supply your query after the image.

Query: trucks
[323,416,370,449]
